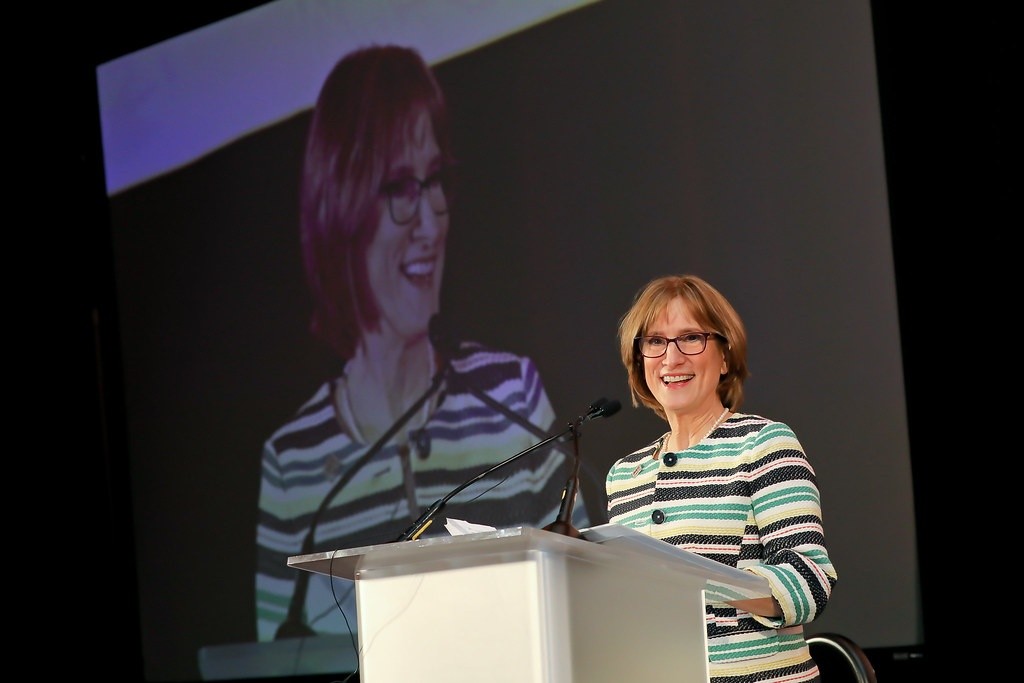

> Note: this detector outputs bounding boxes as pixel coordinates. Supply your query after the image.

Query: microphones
[394,398,623,544]
[274,314,609,641]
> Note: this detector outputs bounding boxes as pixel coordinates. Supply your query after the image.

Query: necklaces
[657,407,729,459]
[336,338,436,443]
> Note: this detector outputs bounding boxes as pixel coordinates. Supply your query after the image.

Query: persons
[605,275,838,683]
[253,46,594,639]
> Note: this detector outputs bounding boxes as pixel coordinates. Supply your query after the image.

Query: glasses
[633,332,716,359]
[382,165,461,225]
